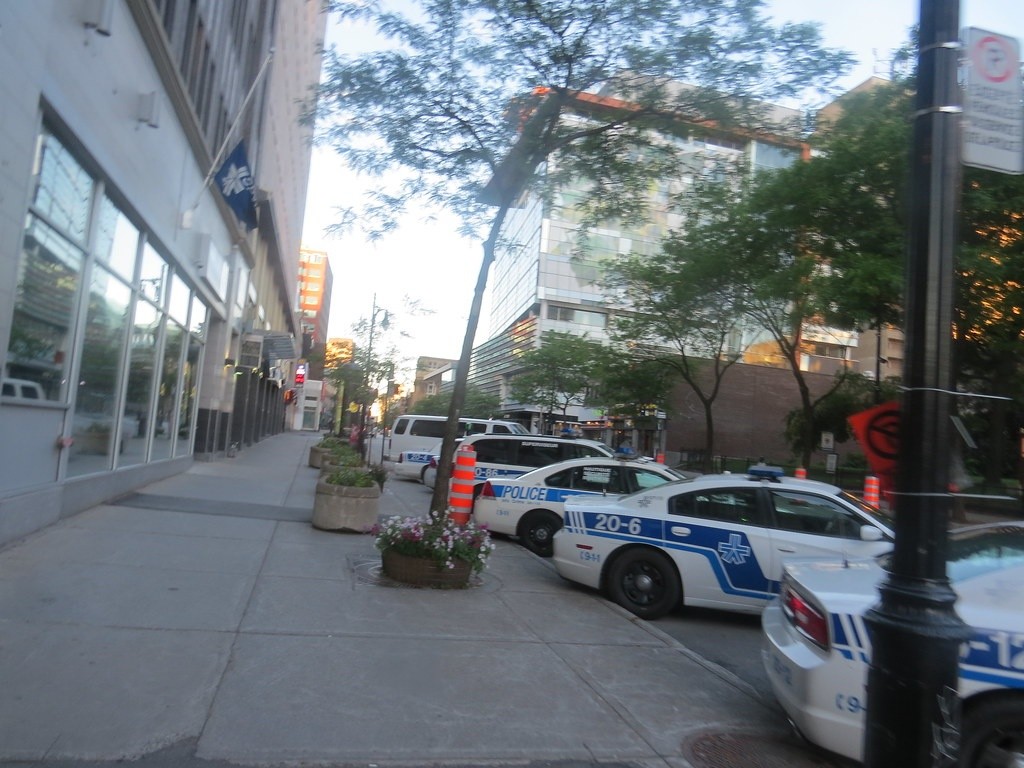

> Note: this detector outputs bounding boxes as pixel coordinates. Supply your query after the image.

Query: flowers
[371,508,495,578]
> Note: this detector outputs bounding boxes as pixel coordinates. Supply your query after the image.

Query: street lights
[358,308,390,451]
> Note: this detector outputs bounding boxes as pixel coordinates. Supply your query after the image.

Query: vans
[452,432,620,499]
[389,414,531,464]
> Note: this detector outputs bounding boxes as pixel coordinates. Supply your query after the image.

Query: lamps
[225,359,235,367]
[235,368,245,374]
[138,90,161,127]
[84,0,114,36]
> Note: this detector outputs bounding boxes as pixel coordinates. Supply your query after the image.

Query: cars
[363,426,384,437]
[762,519,1023,768]
[395,433,464,481]
[552,462,895,619]
[472,448,709,557]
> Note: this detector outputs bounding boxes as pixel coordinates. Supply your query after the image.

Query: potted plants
[309,438,390,533]
[73,422,124,456]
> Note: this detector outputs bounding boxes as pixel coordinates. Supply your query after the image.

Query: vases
[382,547,471,587]
